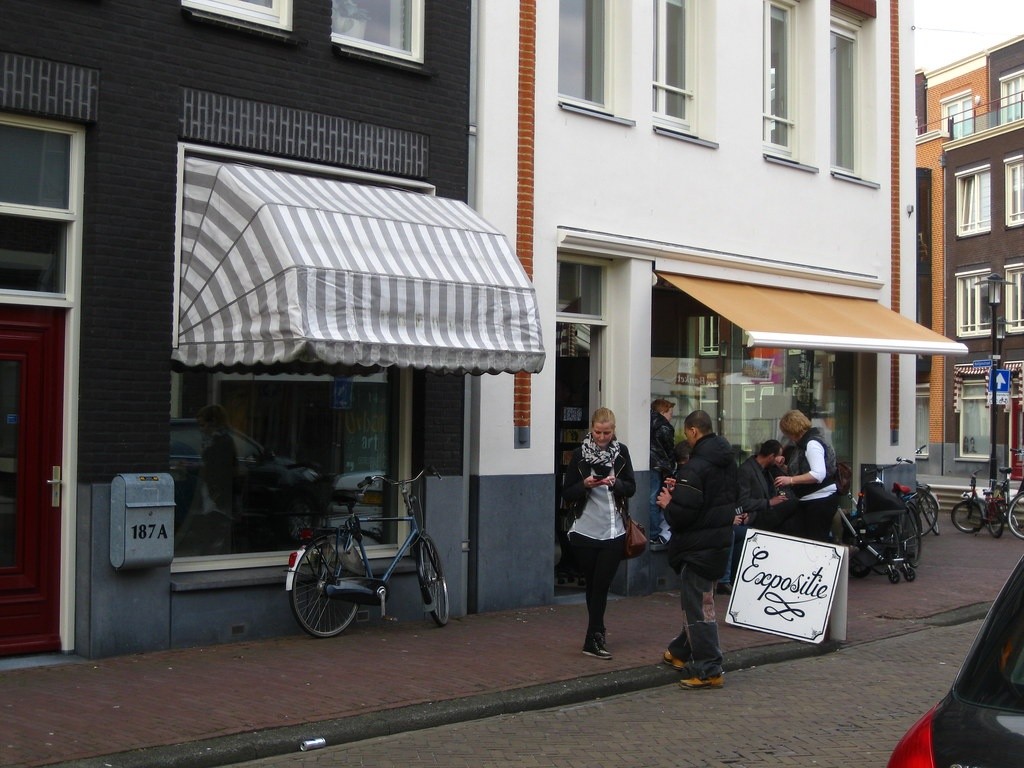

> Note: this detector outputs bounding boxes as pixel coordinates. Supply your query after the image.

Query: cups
[735,506,745,524]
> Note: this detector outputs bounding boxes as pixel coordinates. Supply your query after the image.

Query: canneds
[300,737,326,751]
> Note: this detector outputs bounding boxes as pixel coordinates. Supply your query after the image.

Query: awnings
[657,274,969,356]
[169,155,546,377]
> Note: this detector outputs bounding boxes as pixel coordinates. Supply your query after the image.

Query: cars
[167,415,321,547]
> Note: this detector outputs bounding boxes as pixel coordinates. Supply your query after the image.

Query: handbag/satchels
[836,463,854,495]
[625,516,648,559]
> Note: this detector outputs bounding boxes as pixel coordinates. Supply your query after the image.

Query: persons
[562,407,637,660]
[650,400,844,594]
[175,402,319,556]
[655,410,738,690]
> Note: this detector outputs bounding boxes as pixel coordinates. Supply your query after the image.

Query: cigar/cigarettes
[667,477,672,484]
[609,480,612,484]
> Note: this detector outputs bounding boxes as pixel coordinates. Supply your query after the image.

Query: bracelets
[791,477,793,486]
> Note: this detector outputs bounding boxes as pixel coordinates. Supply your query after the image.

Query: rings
[780,482,782,485]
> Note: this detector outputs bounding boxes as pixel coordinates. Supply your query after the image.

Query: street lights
[974,272,1017,489]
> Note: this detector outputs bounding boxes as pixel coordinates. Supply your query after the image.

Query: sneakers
[581,630,613,660]
[680,674,725,689]
[663,650,686,670]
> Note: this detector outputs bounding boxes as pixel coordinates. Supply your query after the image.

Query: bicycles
[950,469,1006,539]
[285,464,450,638]
[985,447,1024,539]
[856,441,942,576]
[295,479,384,547]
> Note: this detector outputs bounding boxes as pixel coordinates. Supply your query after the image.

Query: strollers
[834,480,917,585]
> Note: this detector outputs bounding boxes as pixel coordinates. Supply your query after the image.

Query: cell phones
[593,475,603,481]
